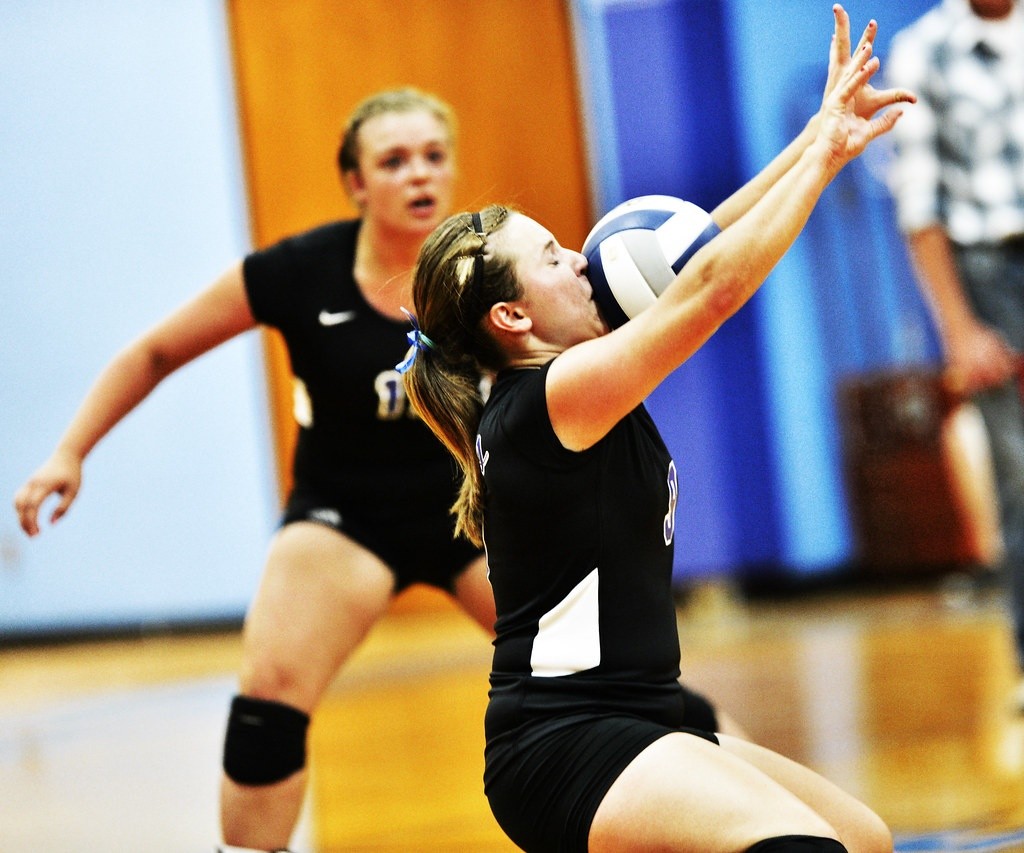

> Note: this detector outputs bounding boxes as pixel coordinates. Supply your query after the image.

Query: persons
[14,85,496,853]
[396,0,917,853]
[886,0,1024,702]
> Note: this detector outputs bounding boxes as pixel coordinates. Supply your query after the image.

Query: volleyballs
[576,191,727,334]
